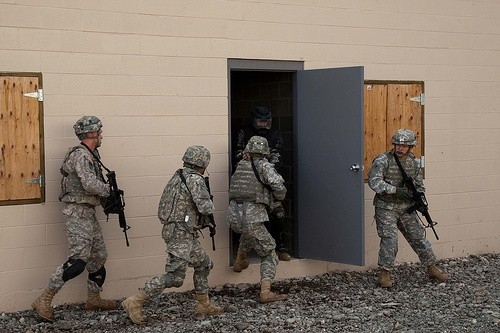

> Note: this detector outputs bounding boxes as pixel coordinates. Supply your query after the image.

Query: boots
[427,261,448,283]
[30,288,58,322]
[379,270,392,288]
[233,249,249,273]
[260,279,288,303]
[85,286,117,310]
[195,290,223,319]
[121,289,149,325]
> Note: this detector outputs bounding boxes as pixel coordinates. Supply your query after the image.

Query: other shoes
[277,253,291,261]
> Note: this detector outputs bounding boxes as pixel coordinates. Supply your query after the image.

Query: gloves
[395,187,410,201]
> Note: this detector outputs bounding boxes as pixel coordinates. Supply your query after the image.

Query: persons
[31,116,117,320]
[368,128,451,288]
[122,145,222,323]
[233,105,291,261]
[226,136,289,302]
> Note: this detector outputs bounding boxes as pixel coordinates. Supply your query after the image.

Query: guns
[204,176,216,251]
[100,169,131,247]
[393,152,440,240]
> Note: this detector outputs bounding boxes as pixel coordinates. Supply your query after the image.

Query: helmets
[244,136,270,155]
[251,105,272,135]
[182,145,210,168]
[73,116,103,134]
[391,129,417,147]
